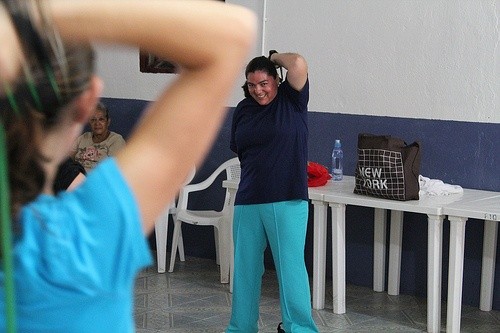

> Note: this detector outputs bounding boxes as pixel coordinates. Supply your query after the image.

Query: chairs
[154,161,196,273]
[168,156,241,285]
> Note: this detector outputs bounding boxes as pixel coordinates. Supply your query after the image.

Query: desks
[444,195,500,333]
[221,173,356,310]
[323,182,500,333]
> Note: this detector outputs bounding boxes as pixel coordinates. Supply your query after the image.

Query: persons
[72,100,129,177]
[226,50,318,333]
[0,0,258,333]
[51,156,87,198]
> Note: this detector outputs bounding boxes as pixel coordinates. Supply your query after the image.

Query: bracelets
[268,50,281,69]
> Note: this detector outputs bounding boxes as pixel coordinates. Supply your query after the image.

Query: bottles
[331,140,344,181]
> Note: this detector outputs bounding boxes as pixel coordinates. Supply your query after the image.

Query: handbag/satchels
[242,51,284,99]
[307,161,331,186]
[353,133,422,201]
